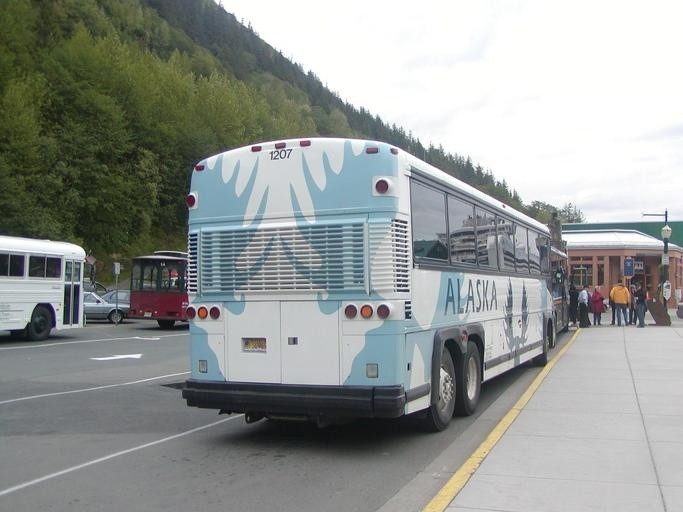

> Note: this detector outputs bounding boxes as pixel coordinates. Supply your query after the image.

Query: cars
[83,291,130,324]
[84,279,107,296]
[101,289,131,305]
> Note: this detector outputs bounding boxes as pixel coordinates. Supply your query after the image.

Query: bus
[0,234,87,341]
[128,255,190,329]
[181,136,552,433]
[551,245,570,349]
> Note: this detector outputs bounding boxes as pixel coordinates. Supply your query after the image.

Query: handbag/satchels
[603,304,608,313]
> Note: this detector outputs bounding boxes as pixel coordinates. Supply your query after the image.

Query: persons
[563,278,665,329]
